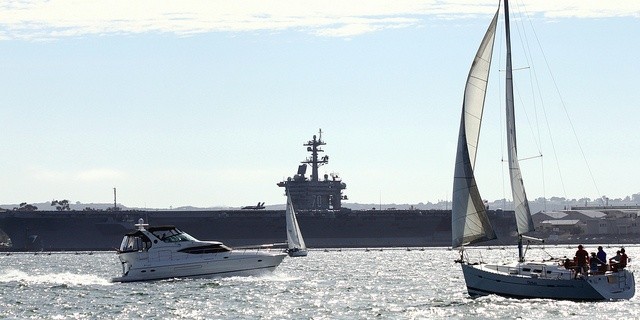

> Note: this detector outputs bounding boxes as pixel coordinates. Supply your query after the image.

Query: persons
[596,246,606,263]
[588,252,606,274]
[574,244,589,278]
[564,258,572,269]
[609,250,621,272]
[615,248,629,273]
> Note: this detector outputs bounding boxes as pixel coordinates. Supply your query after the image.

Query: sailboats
[452,0,635,302]
[285,183,308,258]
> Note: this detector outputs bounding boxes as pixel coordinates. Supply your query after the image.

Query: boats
[110,219,289,283]
